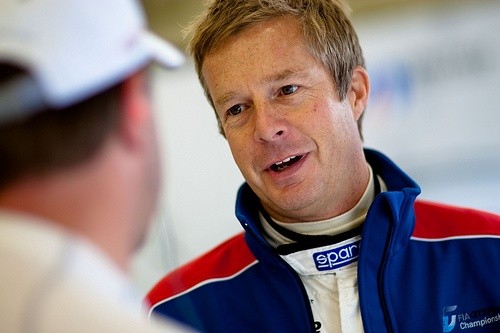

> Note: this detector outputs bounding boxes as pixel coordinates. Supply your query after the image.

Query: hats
[0,1,186,118]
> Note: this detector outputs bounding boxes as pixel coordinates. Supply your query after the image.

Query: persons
[143,0,500,333]
[0,0,200,333]
